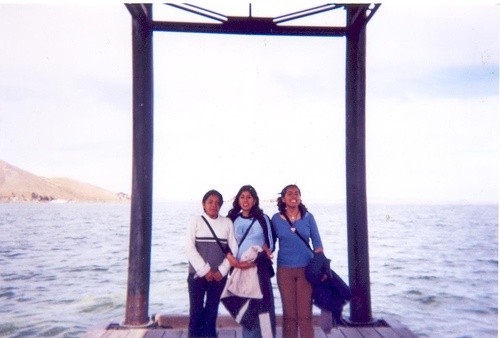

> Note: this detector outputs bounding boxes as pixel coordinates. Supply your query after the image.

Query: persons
[225,184,277,338]
[185,190,238,338]
[262,185,327,338]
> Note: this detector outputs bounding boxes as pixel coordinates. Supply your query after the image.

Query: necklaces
[285,209,300,232]
